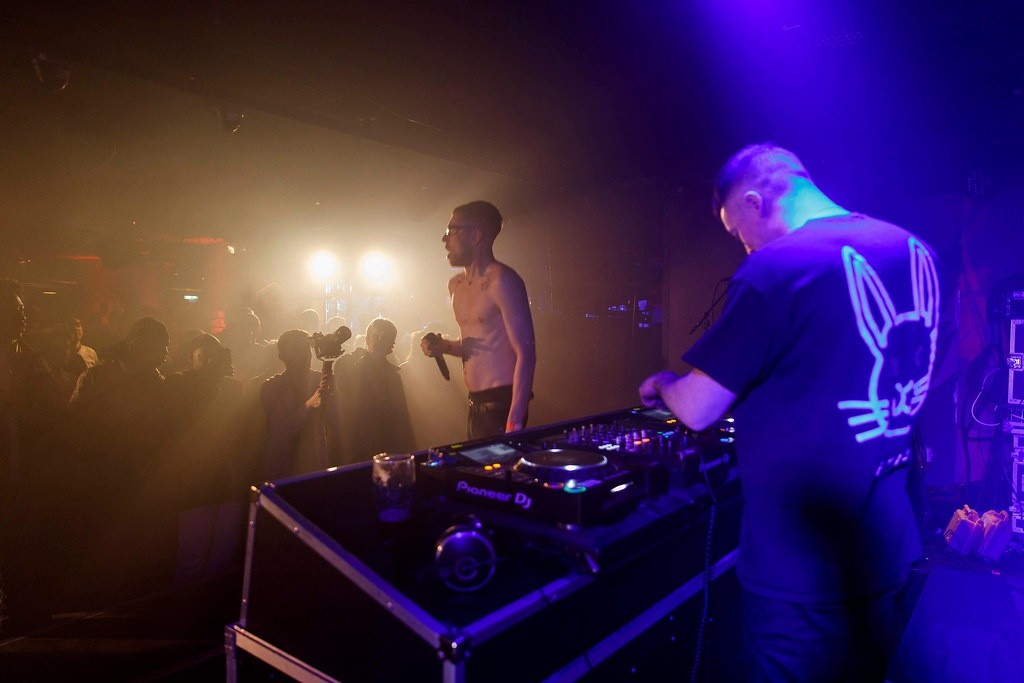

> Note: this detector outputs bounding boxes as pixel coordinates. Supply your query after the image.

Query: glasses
[445,224,475,235]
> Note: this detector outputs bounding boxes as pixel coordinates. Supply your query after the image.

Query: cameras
[310,325,352,359]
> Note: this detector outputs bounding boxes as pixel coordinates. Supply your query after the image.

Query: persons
[638,145,938,683]
[0,277,407,629]
[421,202,536,443]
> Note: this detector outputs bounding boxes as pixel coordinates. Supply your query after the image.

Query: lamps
[210,97,245,136]
[26,55,73,97]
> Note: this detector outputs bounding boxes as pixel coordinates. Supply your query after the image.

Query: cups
[372,452,417,523]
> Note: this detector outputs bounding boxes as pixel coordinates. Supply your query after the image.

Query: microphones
[426,333,451,382]
